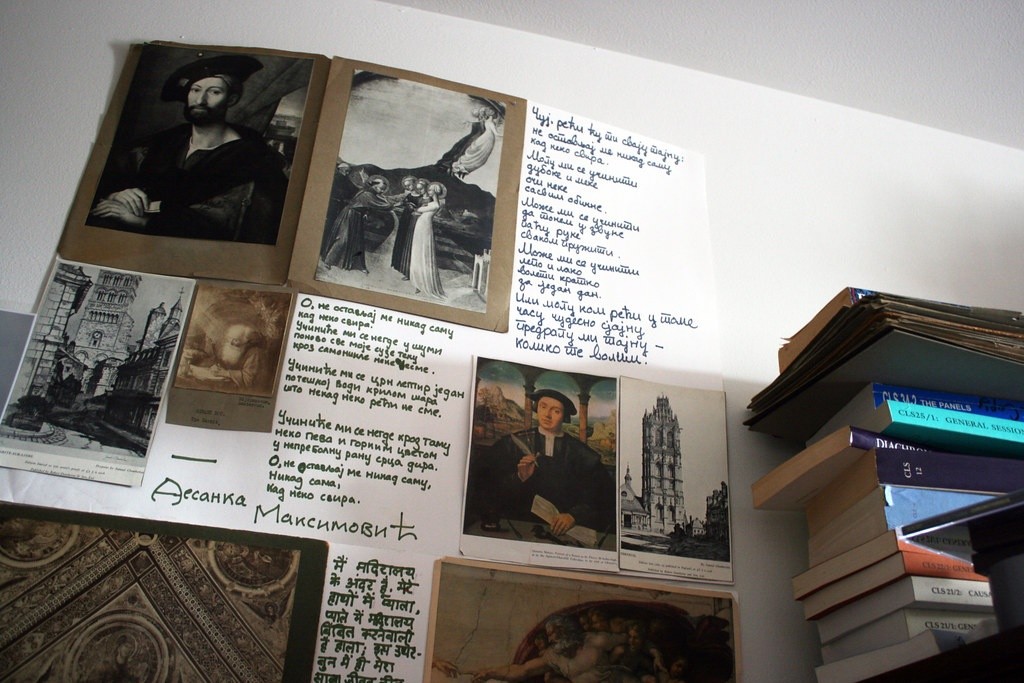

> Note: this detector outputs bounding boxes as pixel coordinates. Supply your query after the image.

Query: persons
[430,605,695,683]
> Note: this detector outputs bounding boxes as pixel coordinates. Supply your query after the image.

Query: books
[742,286,1024,683]
